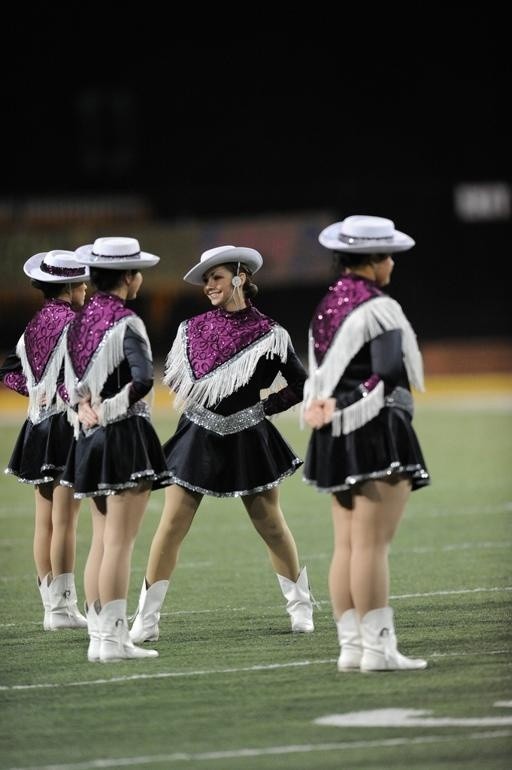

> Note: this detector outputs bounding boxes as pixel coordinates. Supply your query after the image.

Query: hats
[316,213,415,255]
[184,241,264,288]
[21,246,91,286]
[74,235,162,271]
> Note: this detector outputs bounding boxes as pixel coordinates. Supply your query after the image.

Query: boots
[81,595,161,664]
[32,568,88,630]
[126,573,170,645]
[363,607,431,675]
[333,609,364,671]
[272,563,318,634]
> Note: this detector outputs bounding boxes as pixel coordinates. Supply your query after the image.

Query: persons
[0,247,94,631]
[56,236,174,663]
[300,213,430,674]
[128,243,314,645]
